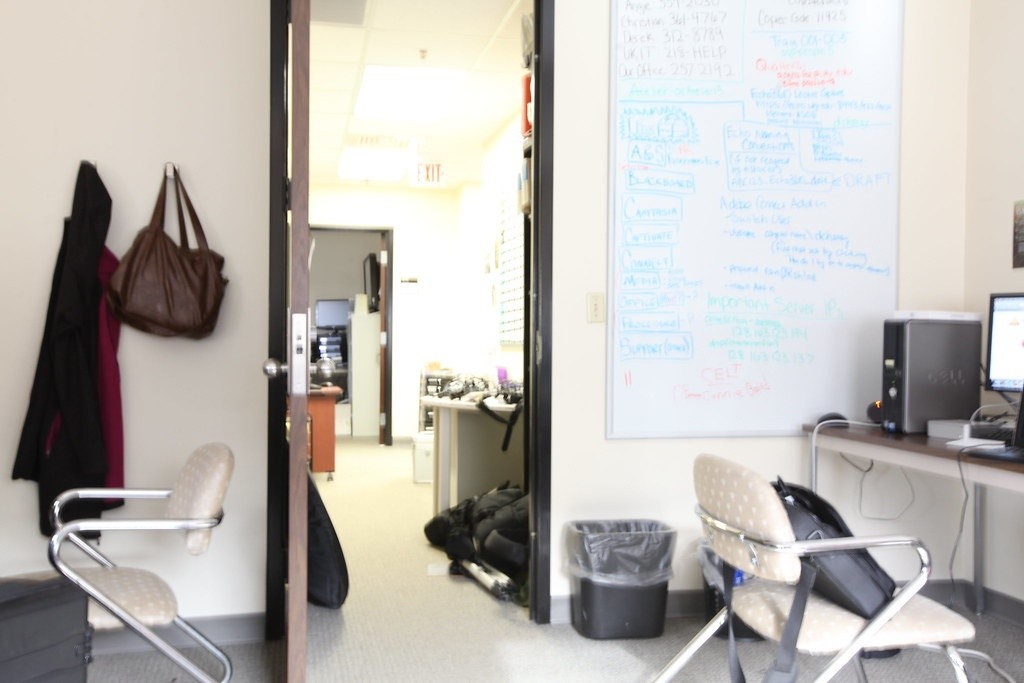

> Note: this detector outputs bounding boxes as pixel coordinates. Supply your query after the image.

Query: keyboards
[968,447,1024,463]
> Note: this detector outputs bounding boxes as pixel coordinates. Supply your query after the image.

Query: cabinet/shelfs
[0,577,95,683]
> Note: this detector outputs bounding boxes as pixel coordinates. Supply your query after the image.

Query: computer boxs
[882,319,982,434]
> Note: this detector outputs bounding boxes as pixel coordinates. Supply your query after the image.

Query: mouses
[816,413,849,428]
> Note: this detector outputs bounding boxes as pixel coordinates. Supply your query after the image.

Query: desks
[802,422,1024,616]
[429,400,524,520]
[284,385,344,482]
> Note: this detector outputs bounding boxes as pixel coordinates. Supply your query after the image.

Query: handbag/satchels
[106,162,229,340]
[724,475,896,683]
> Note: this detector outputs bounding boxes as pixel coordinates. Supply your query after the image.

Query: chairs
[646,452,977,683]
[6,442,235,683]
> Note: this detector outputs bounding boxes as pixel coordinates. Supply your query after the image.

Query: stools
[411,431,434,484]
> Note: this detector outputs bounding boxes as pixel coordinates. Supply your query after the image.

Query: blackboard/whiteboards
[602,1,909,444]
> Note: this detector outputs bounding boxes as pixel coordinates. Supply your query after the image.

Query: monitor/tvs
[984,293,1024,393]
[315,300,350,330]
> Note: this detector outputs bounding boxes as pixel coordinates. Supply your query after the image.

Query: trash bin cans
[567,518,678,641]
[704,540,764,642]
